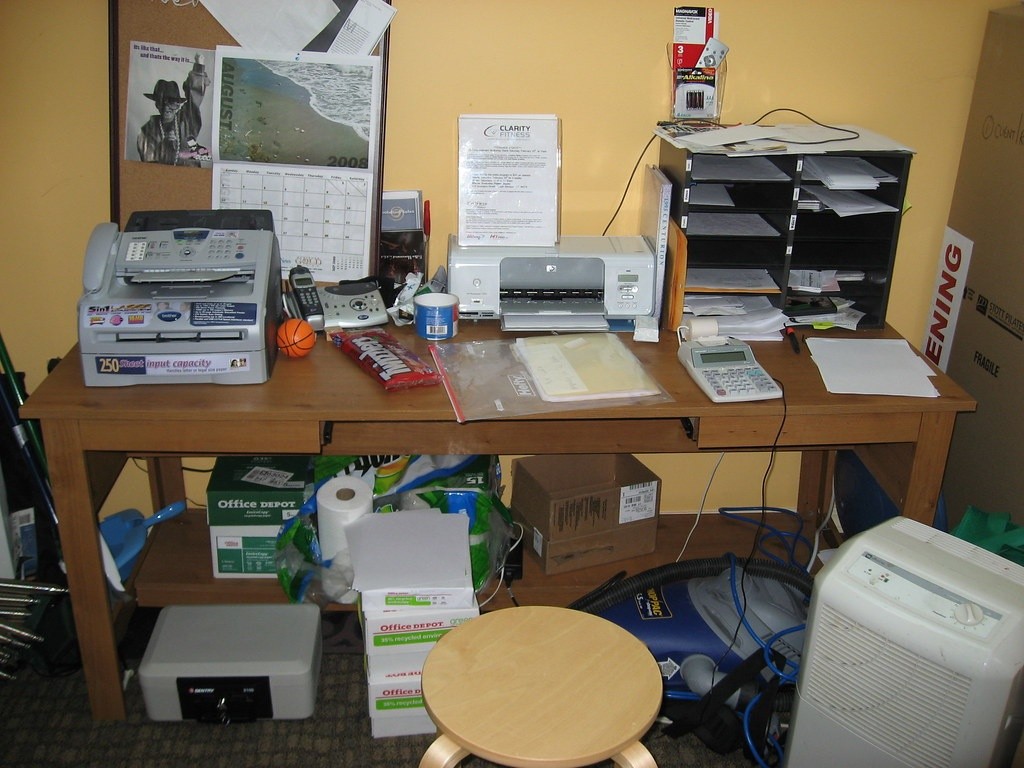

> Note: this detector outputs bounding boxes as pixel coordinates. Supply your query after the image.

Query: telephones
[287,263,388,329]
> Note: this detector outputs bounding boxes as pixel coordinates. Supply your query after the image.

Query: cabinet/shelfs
[660,120,912,331]
[19,318,978,726]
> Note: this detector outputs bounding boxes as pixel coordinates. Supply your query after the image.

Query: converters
[497,538,523,580]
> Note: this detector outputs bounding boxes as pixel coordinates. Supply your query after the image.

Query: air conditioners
[785,516,1024,768]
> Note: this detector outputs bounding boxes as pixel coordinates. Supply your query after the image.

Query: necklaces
[157,113,181,166]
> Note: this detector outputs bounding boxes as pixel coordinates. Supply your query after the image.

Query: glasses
[155,101,180,110]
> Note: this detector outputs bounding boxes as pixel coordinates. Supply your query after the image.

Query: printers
[138,601,323,720]
[81,208,282,387]
[447,233,656,331]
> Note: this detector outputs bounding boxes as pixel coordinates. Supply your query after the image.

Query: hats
[143,79,188,103]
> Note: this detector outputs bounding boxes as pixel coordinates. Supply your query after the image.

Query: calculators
[680,340,784,402]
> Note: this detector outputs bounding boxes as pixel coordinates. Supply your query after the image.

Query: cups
[413,293,460,340]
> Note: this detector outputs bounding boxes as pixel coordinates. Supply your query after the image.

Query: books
[638,163,672,318]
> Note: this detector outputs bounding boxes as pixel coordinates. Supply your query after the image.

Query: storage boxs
[358,582,481,739]
[207,456,311,579]
[511,452,661,574]
[836,2,1024,566]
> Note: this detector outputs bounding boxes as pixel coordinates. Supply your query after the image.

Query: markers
[787,327,801,353]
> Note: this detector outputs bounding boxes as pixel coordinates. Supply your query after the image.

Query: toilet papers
[315,474,375,561]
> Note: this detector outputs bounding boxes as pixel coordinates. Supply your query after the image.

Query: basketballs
[278,319,316,359]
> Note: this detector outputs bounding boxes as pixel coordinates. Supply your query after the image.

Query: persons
[137,78,193,166]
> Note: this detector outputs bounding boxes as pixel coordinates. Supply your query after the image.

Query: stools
[420,605,662,768]
